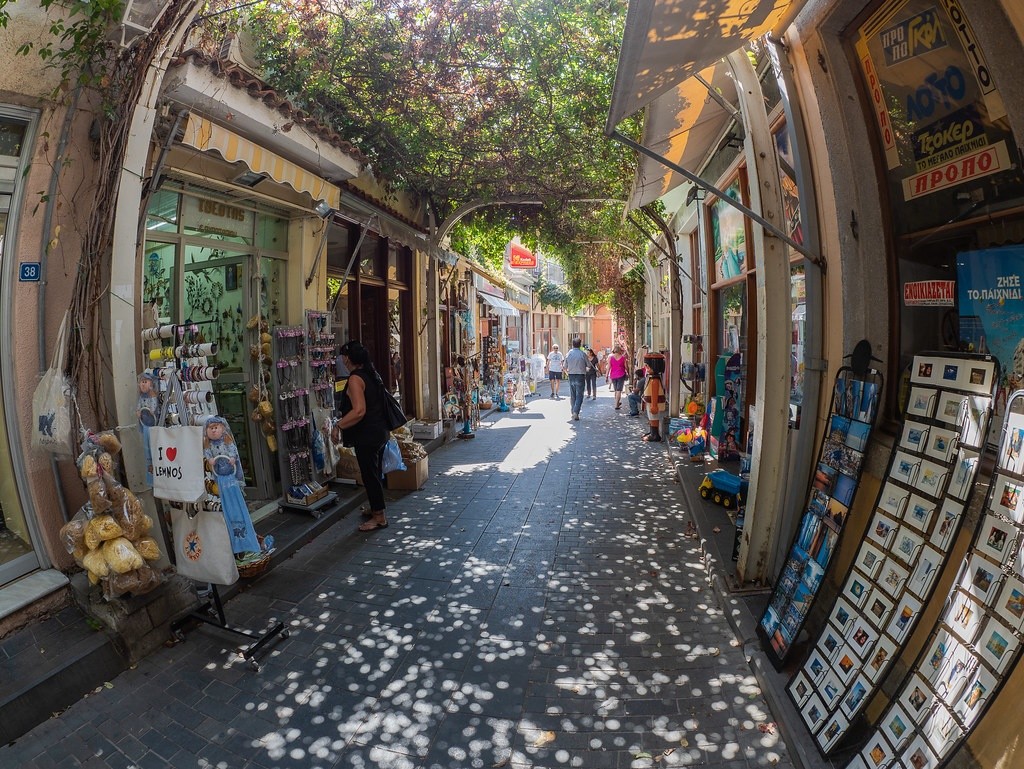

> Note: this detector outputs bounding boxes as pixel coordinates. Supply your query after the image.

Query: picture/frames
[225,264,237,291]
[237,265,243,287]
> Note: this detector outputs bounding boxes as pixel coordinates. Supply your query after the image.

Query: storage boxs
[336,450,366,486]
[284,483,330,505]
[412,421,439,438]
[386,453,428,489]
[405,416,415,429]
[417,418,443,434]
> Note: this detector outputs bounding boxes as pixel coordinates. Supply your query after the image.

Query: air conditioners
[219,14,280,89]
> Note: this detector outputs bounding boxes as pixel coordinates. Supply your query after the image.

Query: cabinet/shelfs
[708,352,741,462]
[212,383,257,487]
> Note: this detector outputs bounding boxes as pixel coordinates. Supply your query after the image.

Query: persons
[547,344,564,398]
[641,352,666,442]
[584,347,603,400]
[393,351,402,398]
[988,531,1004,549]
[335,340,390,532]
[137,368,162,427]
[626,369,645,416]
[202,416,261,553]
[606,346,629,409]
[564,337,592,420]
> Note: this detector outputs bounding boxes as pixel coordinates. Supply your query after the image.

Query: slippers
[362,509,372,515]
[359,521,388,532]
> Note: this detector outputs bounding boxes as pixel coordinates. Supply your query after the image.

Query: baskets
[237,535,273,577]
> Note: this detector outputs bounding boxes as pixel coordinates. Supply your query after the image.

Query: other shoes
[550,393,554,398]
[587,394,590,398]
[572,412,579,420]
[629,410,639,417]
[556,394,559,398]
[593,395,596,400]
[615,402,622,409]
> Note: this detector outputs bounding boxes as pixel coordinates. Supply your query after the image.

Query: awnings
[477,292,520,317]
[310,189,460,335]
[602,0,820,295]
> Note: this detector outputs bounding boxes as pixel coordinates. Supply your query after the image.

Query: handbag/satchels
[381,440,407,475]
[375,371,408,431]
[596,369,601,378]
[147,373,208,503]
[30,310,75,455]
[170,501,240,586]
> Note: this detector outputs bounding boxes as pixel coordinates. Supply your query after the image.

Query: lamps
[311,199,333,220]
[224,161,269,189]
[718,131,744,151]
[117,0,171,56]
[686,184,706,205]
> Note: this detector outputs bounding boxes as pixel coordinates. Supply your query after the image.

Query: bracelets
[337,421,343,430]
